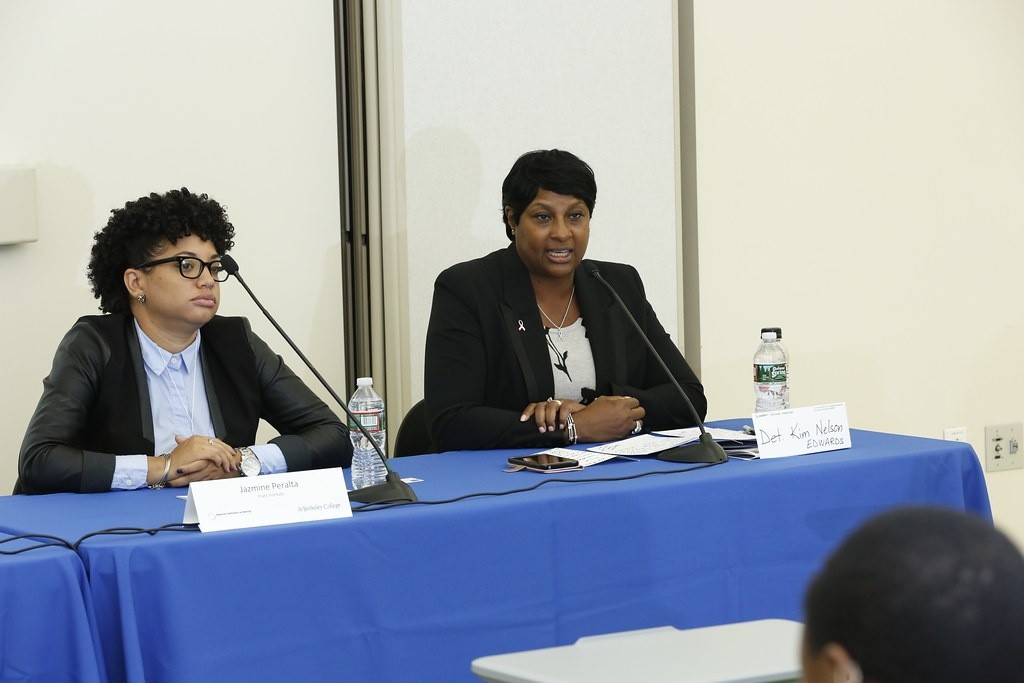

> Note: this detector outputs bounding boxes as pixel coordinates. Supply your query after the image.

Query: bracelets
[148,452,171,490]
[567,412,577,445]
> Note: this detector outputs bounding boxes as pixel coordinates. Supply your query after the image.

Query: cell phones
[508,453,580,470]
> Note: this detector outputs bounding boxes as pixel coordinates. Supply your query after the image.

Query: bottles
[347,377,384,490]
[752,327,791,412]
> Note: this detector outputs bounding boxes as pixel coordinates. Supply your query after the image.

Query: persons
[802,501,1024,681]
[11,186,354,494]
[393,153,707,459]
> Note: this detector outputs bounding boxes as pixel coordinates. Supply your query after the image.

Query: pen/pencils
[743,425,755,435]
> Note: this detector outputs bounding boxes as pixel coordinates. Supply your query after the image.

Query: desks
[0,419,999,683]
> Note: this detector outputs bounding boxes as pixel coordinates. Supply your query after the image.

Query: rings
[207,438,213,445]
[632,421,642,435]
[546,397,561,406]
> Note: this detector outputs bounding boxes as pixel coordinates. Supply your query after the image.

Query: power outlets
[943,427,968,445]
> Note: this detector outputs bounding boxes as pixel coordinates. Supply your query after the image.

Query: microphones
[582,261,731,464]
[220,254,419,505]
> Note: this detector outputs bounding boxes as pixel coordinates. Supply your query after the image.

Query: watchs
[235,446,261,477]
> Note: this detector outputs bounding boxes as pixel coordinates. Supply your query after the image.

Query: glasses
[133,256,230,282]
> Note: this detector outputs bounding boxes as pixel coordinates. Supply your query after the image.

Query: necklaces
[158,334,199,437]
[537,282,576,340]
